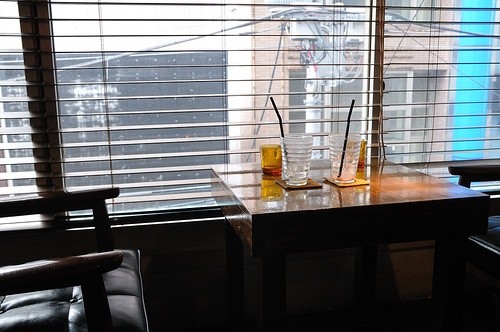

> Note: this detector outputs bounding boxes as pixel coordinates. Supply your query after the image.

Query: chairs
[431,161,500,332]
[0,186,152,332]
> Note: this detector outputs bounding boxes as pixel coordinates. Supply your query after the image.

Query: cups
[259,144,282,175]
[328,131,366,183]
[281,134,314,186]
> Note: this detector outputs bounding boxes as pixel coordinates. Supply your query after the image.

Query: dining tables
[208,155,491,332]
